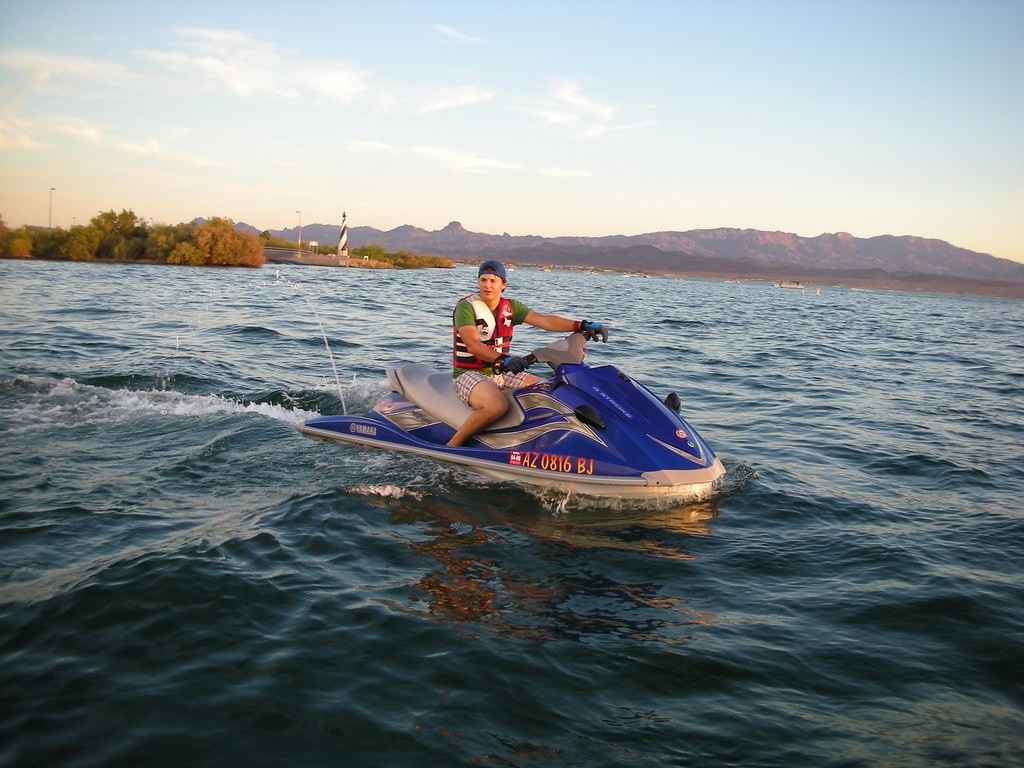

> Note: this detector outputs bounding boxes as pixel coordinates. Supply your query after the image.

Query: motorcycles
[297,325,731,505]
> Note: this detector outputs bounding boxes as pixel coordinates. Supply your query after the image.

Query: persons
[446,261,608,448]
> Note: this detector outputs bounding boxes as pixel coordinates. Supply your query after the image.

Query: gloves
[580,319,608,343]
[492,353,530,376]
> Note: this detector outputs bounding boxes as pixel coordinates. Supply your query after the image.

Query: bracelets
[573,321,580,334]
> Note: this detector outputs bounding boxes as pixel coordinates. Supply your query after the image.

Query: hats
[477,260,507,282]
